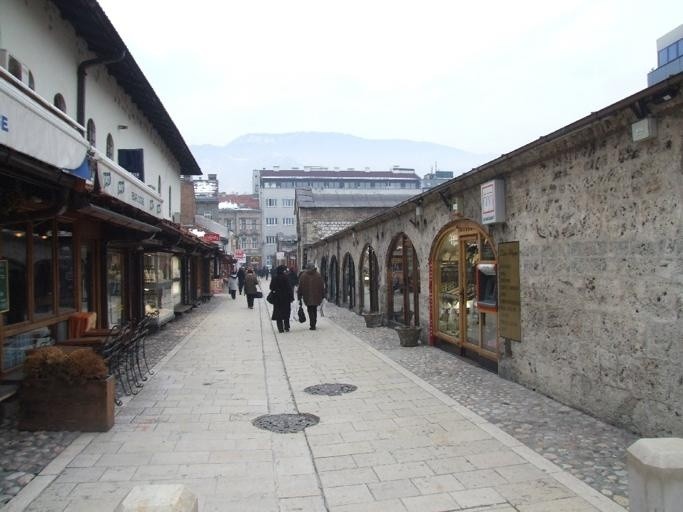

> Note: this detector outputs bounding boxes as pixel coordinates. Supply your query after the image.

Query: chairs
[58,309,160,407]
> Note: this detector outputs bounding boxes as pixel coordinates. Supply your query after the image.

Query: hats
[276,265,287,274]
[303,263,317,272]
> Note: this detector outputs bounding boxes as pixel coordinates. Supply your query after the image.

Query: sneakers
[310,326,315,330]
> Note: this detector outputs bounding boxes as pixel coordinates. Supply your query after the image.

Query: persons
[222,264,304,311]
[267,263,295,335]
[295,262,326,332]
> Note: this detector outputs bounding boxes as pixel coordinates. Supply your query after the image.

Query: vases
[394,326,421,347]
[362,314,381,328]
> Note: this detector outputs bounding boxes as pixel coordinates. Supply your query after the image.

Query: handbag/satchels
[298,299,306,323]
[253,284,263,298]
[267,288,279,304]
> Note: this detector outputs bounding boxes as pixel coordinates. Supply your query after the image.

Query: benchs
[201,292,212,302]
[174,304,193,315]
